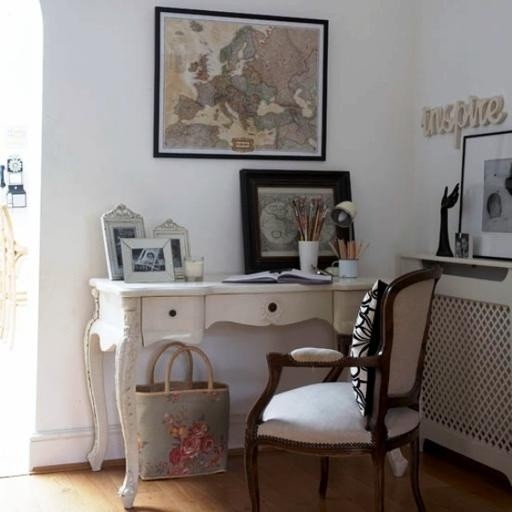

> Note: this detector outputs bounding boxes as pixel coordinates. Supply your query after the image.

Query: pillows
[348,277,389,420]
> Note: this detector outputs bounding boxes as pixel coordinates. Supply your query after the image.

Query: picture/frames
[153,6,329,161]
[99,201,191,284]
[458,130,512,262]
[239,168,355,275]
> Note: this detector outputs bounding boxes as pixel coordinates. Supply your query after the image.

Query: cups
[454,232,469,258]
[331,258,359,278]
[183,256,204,281]
[297,240,318,274]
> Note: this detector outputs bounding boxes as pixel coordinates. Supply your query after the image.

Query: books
[222,268,332,284]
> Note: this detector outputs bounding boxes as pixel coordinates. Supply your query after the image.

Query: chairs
[243,262,445,512]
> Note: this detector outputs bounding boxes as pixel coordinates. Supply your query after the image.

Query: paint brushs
[291,198,332,240]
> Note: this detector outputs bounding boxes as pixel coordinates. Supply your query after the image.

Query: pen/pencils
[328,238,371,259]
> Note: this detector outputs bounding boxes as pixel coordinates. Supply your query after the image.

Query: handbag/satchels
[136,341,231,480]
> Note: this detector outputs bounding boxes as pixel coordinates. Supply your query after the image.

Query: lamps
[329,201,359,242]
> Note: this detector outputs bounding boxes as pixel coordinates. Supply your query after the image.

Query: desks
[81,274,394,509]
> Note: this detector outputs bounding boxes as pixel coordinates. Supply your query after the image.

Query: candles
[185,259,203,277]
